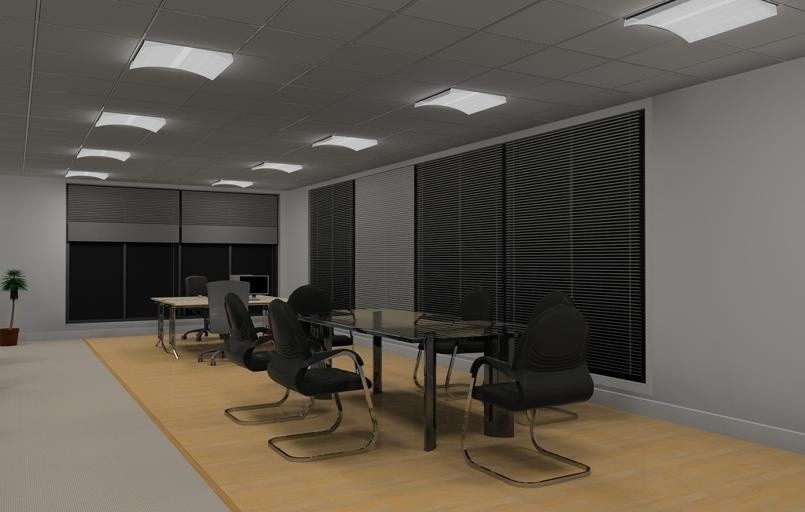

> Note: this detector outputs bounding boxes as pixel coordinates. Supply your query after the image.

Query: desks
[149,294,288,359]
[294,306,519,452]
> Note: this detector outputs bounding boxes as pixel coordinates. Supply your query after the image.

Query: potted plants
[0,268,31,345]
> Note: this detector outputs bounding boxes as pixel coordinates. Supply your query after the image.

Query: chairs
[179,280,356,373]
[461,292,594,486]
[224,298,380,462]
[413,287,495,400]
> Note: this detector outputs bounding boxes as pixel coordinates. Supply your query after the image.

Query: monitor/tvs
[240,275,270,301]
